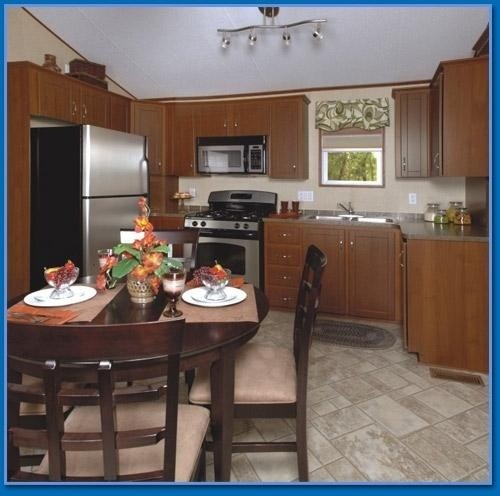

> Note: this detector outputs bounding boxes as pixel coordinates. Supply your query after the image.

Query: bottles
[42,54,61,74]
[423,201,440,223]
[447,201,464,222]
[434,209,449,226]
[423,222,472,236]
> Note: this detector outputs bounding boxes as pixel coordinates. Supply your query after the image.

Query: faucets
[337,201,355,214]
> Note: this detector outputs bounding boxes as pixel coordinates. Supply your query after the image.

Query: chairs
[116,230,201,396]
[189,243,327,481]
[8,318,210,481]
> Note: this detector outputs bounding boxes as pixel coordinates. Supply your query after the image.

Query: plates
[181,285,248,309]
[23,285,97,308]
[192,289,238,301]
[35,289,86,300]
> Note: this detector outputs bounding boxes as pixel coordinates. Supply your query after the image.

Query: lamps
[217,7,326,50]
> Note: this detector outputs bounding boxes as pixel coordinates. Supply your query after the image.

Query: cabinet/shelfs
[302,227,396,321]
[398,234,489,374]
[264,222,304,313]
[391,54,488,179]
[165,94,310,180]
[39,67,107,128]
[132,99,165,175]
[106,94,132,133]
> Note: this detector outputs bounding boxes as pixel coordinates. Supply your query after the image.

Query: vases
[125,269,158,304]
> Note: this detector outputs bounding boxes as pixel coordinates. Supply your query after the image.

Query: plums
[209,268,225,280]
[65,260,75,270]
[49,272,56,280]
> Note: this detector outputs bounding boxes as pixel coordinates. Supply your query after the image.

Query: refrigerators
[31,123,151,292]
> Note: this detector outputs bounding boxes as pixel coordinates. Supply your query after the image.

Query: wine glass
[43,267,79,300]
[198,268,231,301]
[161,266,187,319]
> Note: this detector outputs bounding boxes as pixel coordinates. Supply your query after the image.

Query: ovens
[194,227,264,292]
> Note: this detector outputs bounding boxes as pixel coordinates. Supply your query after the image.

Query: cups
[97,248,112,270]
[291,200,299,213]
[169,256,192,280]
[281,201,288,214]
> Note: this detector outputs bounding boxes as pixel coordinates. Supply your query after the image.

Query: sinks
[349,215,397,225]
[303,214,349,223]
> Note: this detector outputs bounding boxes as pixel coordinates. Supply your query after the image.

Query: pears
[214,260,226,275]
[43,266,61,273]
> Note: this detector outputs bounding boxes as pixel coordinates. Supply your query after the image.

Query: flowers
[98,196,181,296]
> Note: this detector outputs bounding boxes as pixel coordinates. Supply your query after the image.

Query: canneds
[447,202,463,223]
[423,204,440,222]
[434,210,448,224]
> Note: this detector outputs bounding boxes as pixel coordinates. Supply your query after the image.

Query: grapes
[186,266,211,288]
[55,266,78,289]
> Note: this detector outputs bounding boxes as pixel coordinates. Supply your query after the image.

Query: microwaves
[195,134,267,176]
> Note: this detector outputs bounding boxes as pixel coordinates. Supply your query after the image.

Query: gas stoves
[183,208,272,234]
